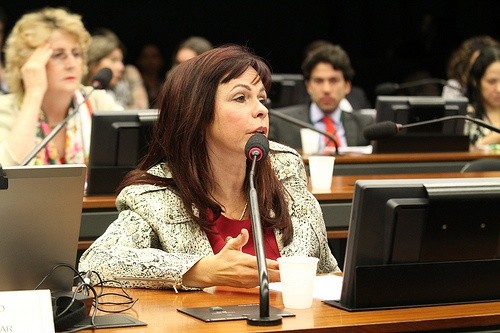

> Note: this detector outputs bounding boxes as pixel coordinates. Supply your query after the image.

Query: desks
[77,149,500,333]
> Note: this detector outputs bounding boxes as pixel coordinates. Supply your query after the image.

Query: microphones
[375,78,468,94]
[244,133,286,327]
[362,115,500,140]
[22,67,113,167]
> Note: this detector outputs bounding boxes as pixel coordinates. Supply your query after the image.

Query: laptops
[0,165,84,297]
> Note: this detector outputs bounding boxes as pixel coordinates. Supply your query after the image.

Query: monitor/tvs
[89,74,500,308]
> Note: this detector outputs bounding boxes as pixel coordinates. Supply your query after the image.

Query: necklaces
[240,201,248,221]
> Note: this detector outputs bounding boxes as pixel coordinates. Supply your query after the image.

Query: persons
[268,45,376,154]
[441,36,500,150]
[77,42,342,293]
[0,8,214,190]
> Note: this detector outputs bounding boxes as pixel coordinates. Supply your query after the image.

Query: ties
[323,116,341,147]
